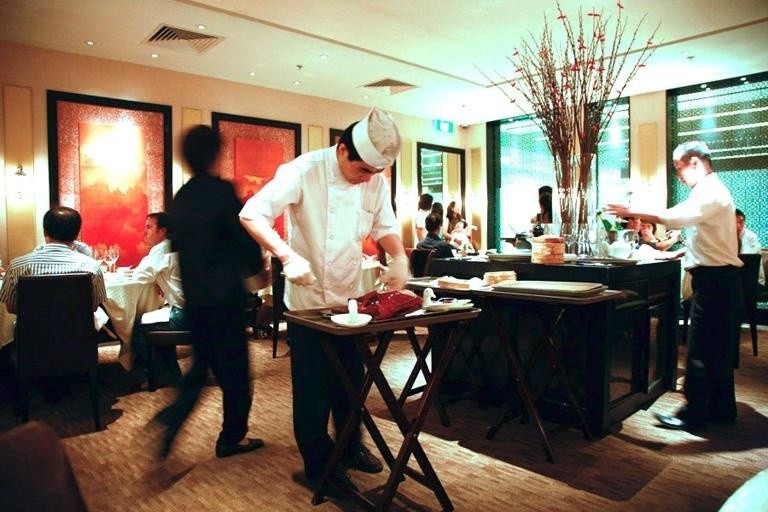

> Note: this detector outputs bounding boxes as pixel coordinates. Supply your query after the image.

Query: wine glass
[87,245,120,274]
[561,222,607,259]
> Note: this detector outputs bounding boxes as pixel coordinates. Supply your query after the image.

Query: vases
[552,154,580,259]
[570,152,599,257]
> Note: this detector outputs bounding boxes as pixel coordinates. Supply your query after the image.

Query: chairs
[270,255,289,359]
[734,253,761,370]
[12,272,103,432]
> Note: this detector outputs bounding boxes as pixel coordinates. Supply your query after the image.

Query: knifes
[279,271,365,294]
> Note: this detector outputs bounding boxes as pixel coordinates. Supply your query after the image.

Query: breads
[480,270,518,287]
[438,276,470,290]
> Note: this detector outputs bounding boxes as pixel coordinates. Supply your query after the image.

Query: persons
[610,217,694,343]
[257,246,284,339]
[144,125,265,457]
[377,195,476,291]
[539,186,552,195]
[238,106,409,496]
[736,211,767,293]
[602,142,744,430]
[530,193,553,225]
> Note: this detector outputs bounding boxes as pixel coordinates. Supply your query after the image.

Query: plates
[590,258,641,265]
[330,299,372,328]
[424,288,473,310]
[488,253,531,262]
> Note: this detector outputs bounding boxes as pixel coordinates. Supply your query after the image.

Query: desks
[429,254,682,442]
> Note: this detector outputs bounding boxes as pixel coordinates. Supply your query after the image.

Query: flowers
[473,0,664,224]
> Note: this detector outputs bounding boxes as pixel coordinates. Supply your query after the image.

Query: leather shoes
[347,444,383,473]
[321,476,358,498]
[215,438,264,457]
[651,411,682,430]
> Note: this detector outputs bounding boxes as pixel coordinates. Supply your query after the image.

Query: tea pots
[601,239,636,258]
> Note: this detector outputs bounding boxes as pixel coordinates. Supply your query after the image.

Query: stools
[148,330,217,384]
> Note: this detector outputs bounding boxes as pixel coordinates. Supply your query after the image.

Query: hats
[351,105,401,170]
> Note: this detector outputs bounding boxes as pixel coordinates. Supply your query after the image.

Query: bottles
[533,214,544,237]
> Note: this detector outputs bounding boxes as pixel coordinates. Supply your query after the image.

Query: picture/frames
[211,110,303,256]
[45,87,175,268]
[329,126,398,219]
